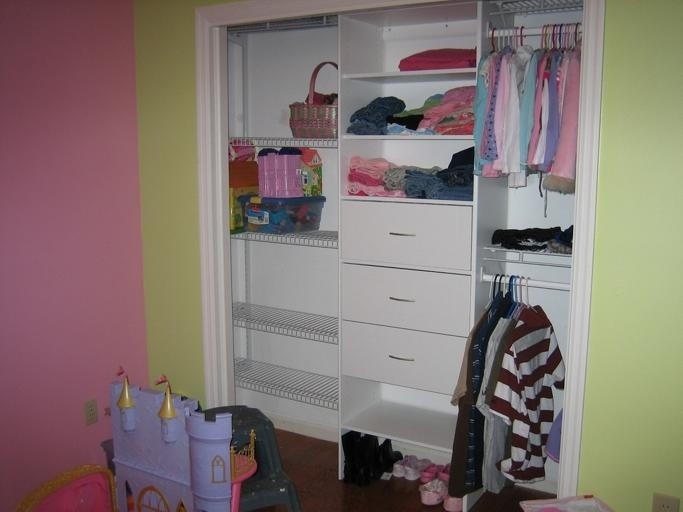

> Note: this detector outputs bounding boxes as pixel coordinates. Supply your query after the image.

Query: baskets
[288,61,338,140]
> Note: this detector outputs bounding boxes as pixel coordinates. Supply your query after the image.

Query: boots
[341,431,402,487]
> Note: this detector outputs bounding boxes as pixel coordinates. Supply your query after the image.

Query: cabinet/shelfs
[193,0,608,512]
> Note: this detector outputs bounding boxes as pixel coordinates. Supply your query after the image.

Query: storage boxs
[235,195,326,236]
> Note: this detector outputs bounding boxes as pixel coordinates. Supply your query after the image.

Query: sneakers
[391,455,462,511]
